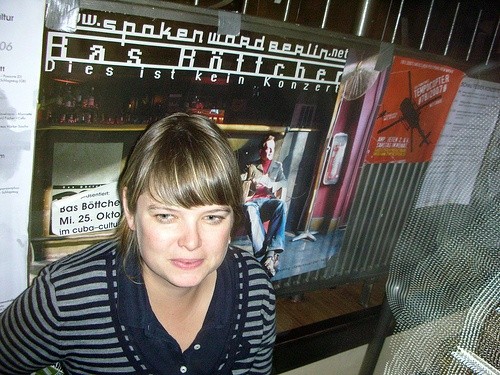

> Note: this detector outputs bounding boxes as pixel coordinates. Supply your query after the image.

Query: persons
[240,135,290,276]
[0,112,276,375]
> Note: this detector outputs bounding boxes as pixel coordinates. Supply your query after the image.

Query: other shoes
[261,256,280,279]
